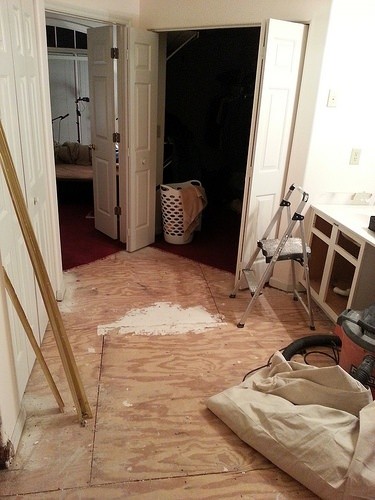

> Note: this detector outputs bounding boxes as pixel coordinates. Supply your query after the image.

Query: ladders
[229,183,318,331]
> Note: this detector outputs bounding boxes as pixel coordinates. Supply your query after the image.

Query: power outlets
[350,148,361,165]
[328,89,338,107]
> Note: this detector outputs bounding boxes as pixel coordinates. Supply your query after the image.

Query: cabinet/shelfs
[300,204,375,328]
[0,0,65,401]
